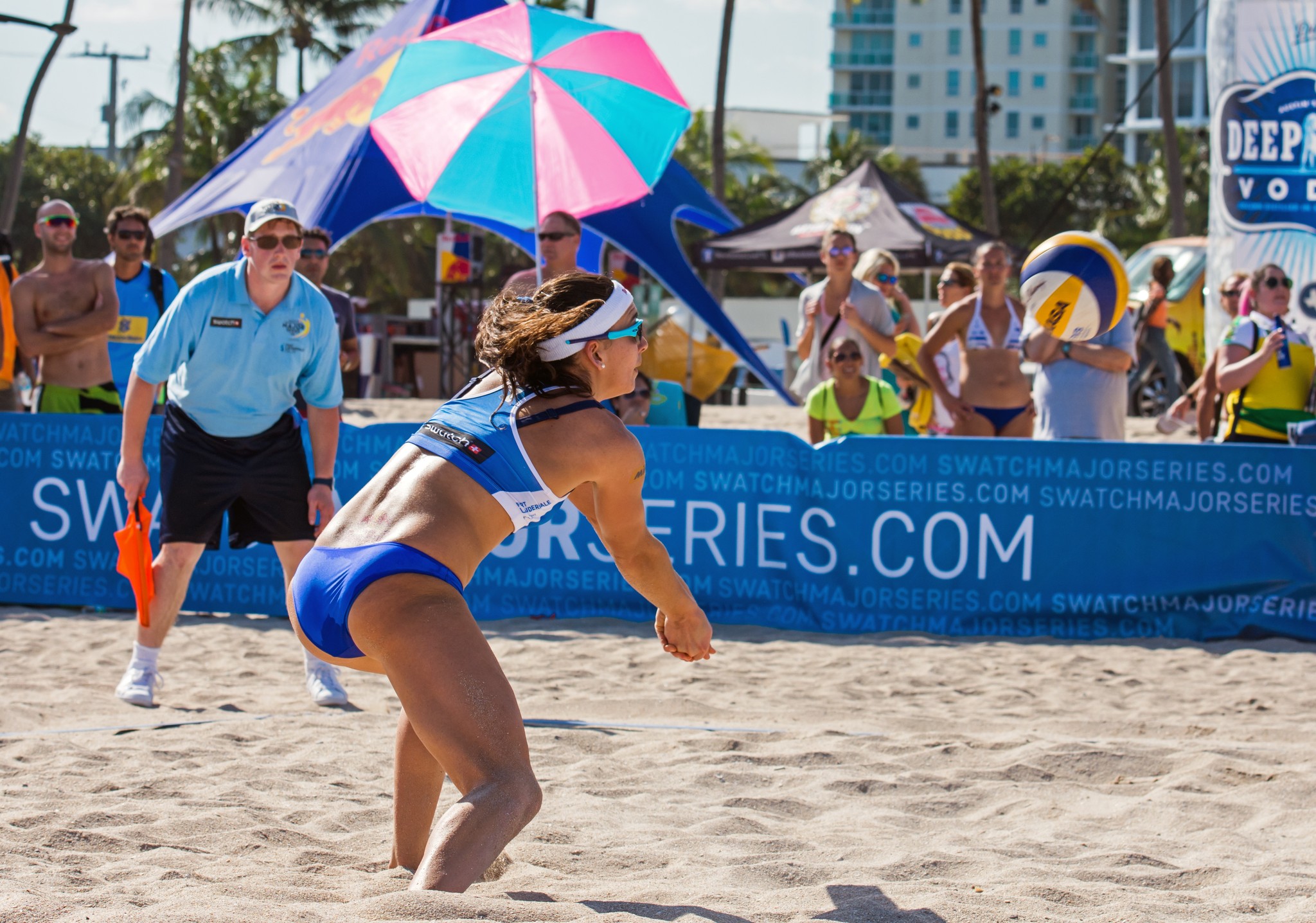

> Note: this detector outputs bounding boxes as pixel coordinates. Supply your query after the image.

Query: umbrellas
[370,0,691,288]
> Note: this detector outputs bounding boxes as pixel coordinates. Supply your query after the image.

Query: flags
[115,494,155,628]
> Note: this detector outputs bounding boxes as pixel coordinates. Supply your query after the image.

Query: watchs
[1063,342,1071,358]
[312,476,335,491]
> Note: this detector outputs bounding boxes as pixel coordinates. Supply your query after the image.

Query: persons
[116,199,348,706]
[287,273,714,894]
[0,199,1315,443]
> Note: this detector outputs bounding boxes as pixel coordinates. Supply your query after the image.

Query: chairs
[599,379,687,427]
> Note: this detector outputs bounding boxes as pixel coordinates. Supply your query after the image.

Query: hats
[244,199,304,239]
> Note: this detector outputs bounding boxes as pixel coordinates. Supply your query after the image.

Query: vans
[1115,237,1213,415]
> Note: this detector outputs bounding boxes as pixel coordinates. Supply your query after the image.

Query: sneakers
[305,662,349,707]
[113,659,164,709]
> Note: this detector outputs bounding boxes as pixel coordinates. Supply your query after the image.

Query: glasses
[624,390,650,399]
[877,274,897,284]
[36,213,80,228]
[300,249,328,259]
[827,246,856,257]
[1222,290,1240,298]
[538,231,575,241]
[248,234,303,250]
[939,278,961,285]
[831,351,861,362]
[565,319,643,343]
[116,229,148,241]
[1262,276,1293,289]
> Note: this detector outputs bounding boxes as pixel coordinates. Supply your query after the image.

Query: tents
[685,160,983,396]
[93,0,808,406]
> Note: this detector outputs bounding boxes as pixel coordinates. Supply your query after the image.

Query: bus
[649,263,948,382]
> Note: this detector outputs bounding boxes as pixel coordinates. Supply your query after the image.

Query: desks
[389,337,483,382]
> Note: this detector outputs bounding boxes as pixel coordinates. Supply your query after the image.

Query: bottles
[1272,314,1290,367]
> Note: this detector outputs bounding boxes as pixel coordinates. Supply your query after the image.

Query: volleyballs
[1019,230,1130,342]
